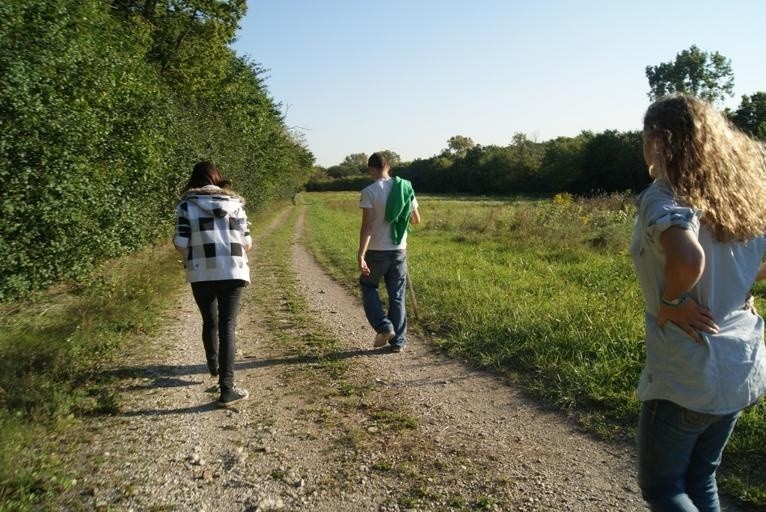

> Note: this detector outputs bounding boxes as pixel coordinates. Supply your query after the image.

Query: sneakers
[216,386,250,409]
[372,328,406,354]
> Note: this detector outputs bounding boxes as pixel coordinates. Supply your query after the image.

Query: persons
[628,93,766,512]
[358,151,420,352]
[172,161,252,407]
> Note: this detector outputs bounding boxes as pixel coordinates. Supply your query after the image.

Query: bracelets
[658,291,689,310]
